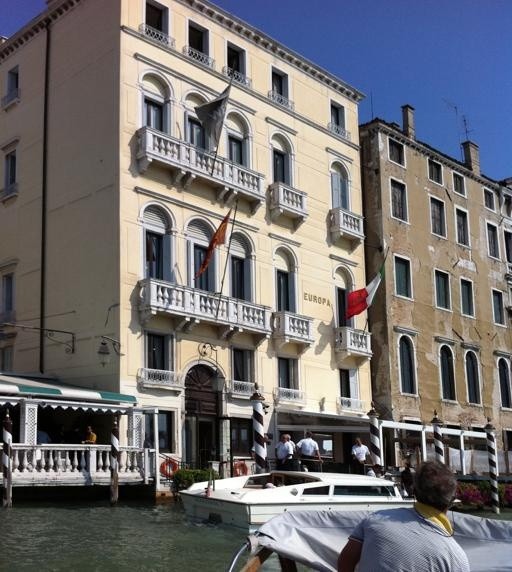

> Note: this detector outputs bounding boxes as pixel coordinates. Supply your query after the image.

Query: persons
[349,436,370,474]
[337,459,470,572]
[367,464,383,477]
[38,425,52,443]
[81,425,97,444]
[275,431,324,471]
[400,463,413,496]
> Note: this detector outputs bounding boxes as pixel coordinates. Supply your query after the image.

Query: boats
[177,471,418,534]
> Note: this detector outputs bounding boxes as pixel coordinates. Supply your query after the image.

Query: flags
[193,204,234,280]
[344,260,384,319]
[194,85,233,153]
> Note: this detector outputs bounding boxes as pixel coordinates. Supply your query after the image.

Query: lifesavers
[233,462,248,476]
[161,459,178,477]
